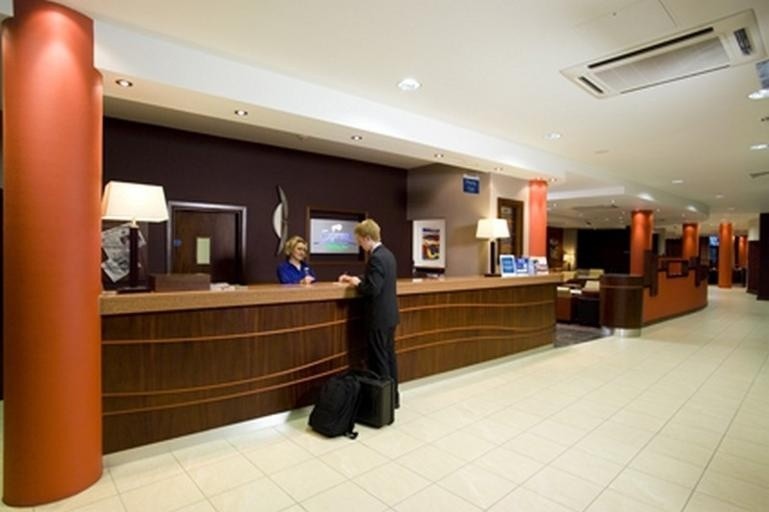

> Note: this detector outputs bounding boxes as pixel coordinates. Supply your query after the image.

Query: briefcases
[348,368,395,429]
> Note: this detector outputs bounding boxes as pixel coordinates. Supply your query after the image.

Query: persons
[339,218,400,409]
[277,235,317,285]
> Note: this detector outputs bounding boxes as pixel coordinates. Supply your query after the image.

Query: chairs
[557,281,601,328]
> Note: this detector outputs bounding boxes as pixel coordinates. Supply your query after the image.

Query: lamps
[476,218,511,277]
[101,180,170,294]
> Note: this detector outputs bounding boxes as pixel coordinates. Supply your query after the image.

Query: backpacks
[309,372,363,439]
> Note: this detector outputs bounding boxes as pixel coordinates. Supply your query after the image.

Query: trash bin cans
[599,274,644,337]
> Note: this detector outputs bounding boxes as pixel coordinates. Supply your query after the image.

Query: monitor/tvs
[309,212,366,261]
[709,235,719,247]
